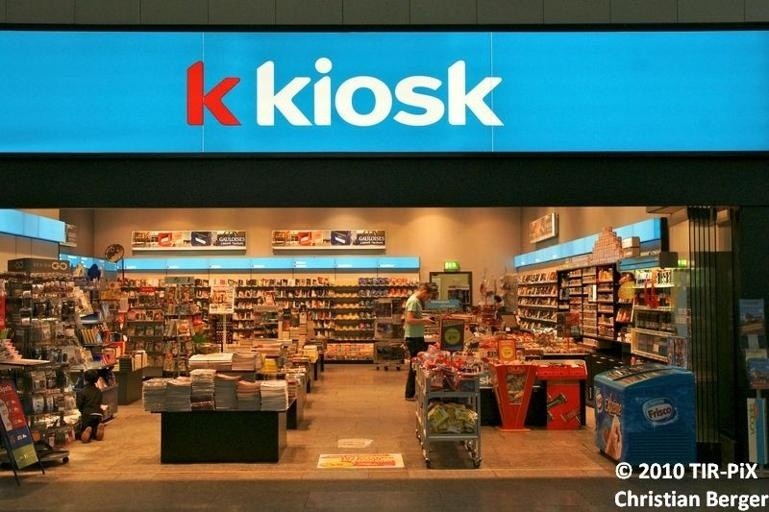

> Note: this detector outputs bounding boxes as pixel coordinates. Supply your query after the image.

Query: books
[142,337,319,413]
[80,328,148,373]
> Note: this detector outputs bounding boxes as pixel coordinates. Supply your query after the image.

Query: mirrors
[105,244,124,263]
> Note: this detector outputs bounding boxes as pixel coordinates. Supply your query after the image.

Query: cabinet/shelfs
[0,255,693,486]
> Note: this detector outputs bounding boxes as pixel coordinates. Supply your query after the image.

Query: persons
[73,370,105,441]
[403,282,436,401]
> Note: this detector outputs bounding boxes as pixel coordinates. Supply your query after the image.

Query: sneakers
[96,423,104,441]
[81,426,93,443]
[407,396,418,400]
